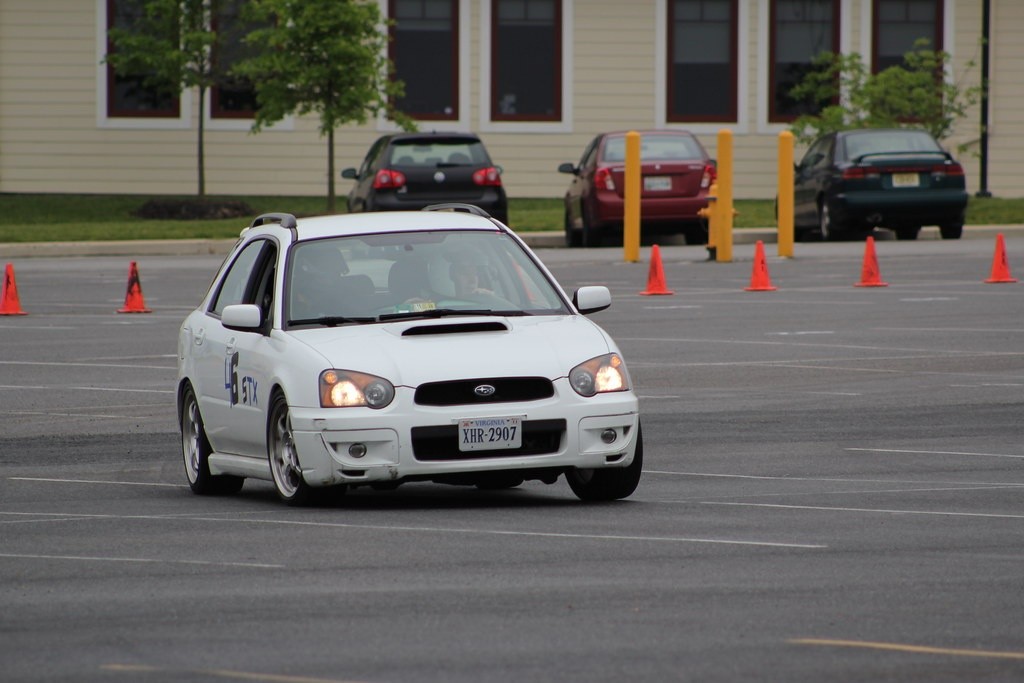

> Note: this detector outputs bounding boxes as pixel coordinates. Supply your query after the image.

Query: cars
[774,126,968,242]
[174,200,646,508]
[557,127,718,248]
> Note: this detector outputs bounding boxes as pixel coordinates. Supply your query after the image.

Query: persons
[406,250,520,309]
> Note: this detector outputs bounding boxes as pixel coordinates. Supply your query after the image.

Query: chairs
[341,255,446,315]
[399,153,473,165]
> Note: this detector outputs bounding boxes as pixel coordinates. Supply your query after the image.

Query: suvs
[340,131,511,233]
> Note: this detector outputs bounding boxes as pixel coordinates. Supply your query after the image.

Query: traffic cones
[0,262,29,315]
[116,259,153,313]
[638,244,675,296]
[854,236,888,286]
[983,233,1019,283]
[743,239,778,291]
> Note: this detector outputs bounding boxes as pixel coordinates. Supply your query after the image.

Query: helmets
[423,244,481,297]
